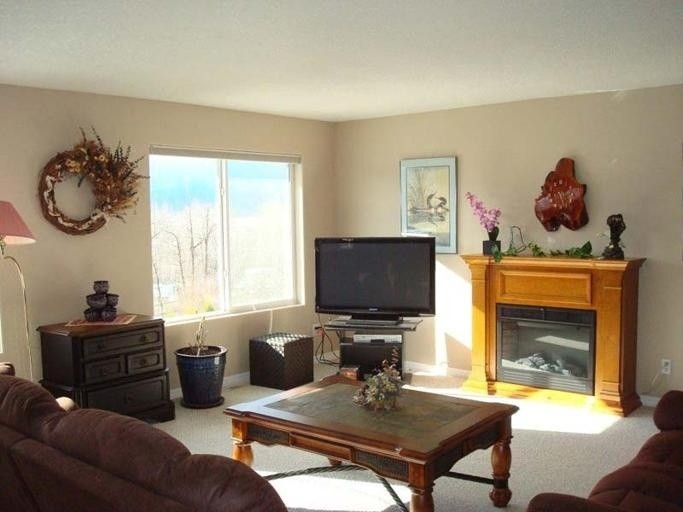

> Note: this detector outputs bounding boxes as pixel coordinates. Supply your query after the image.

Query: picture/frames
[398,155,458,255]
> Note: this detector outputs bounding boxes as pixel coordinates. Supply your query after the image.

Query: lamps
[0,199,38,382]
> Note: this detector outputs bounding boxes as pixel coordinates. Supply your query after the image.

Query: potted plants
[169,316,227,408]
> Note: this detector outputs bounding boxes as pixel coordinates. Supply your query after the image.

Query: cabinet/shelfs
[36,312,175,423]
[324,323,418,380]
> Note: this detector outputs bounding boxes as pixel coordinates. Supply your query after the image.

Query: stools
[250,328,313,390]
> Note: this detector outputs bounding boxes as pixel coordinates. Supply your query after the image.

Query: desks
[222,371,517,511]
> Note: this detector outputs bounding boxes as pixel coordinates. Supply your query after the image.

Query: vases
[487,226,502,252]
[365,394,398,412]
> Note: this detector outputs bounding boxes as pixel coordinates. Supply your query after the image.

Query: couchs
[527,388,681,509]
[0,363,287,510]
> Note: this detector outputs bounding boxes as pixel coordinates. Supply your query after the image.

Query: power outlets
[661,358,670,374]
[313,323,322,337]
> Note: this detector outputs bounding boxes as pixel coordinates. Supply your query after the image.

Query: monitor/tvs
[314,237,436,325]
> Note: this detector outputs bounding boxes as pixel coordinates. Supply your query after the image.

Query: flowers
[350,348,409,409]
[462,192,501,232]
[36,130,145,238]
